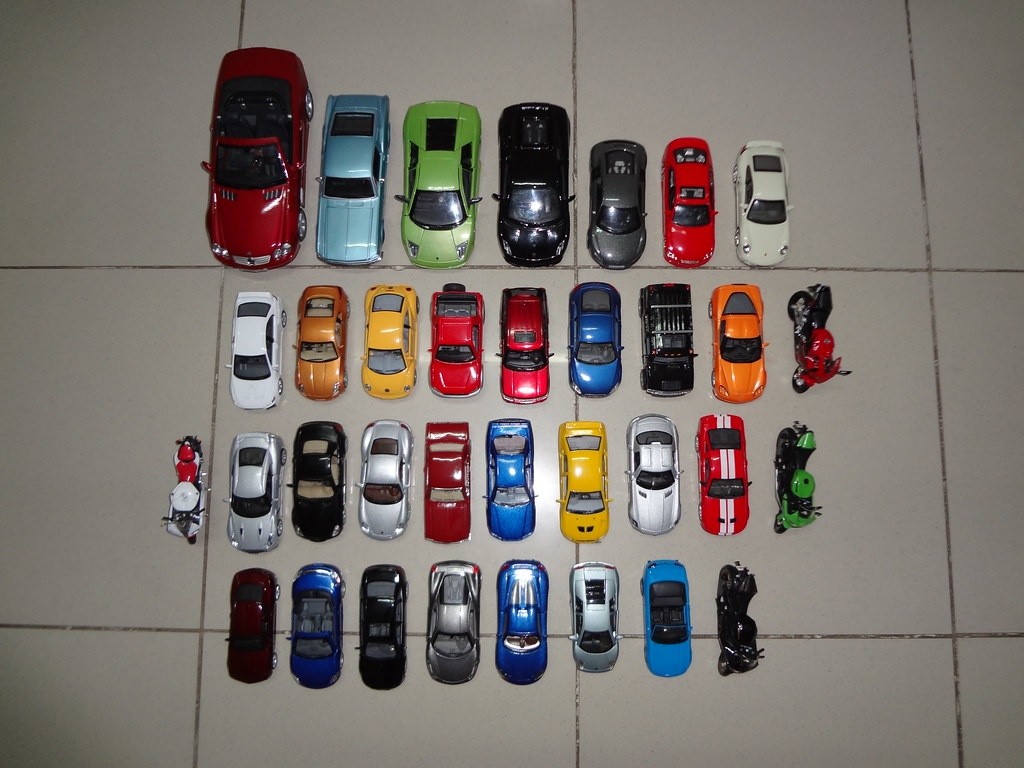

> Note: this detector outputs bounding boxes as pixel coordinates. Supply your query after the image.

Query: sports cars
[640,559,693,678]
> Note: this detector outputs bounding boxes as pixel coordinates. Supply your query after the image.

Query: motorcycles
[715,559,765,677]
[160,436,209,539]
[786,283,853,394]
[774,420,823,534]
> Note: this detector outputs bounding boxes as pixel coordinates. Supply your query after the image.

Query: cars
[482,417,540,542]
[285,563,345,688]
[425,559,483,684]
[222,431,287,555]
[225,565,281,683]
[495,559,548,685]
[568,560,625,674]
[422,420,472,545]
[556,421,615,544]
[354,419,415,542]
[624,412,685,536]
[286,420,349,542]
[694,413,752,534]
[201,47,797,405]
[354,563,407,689]
